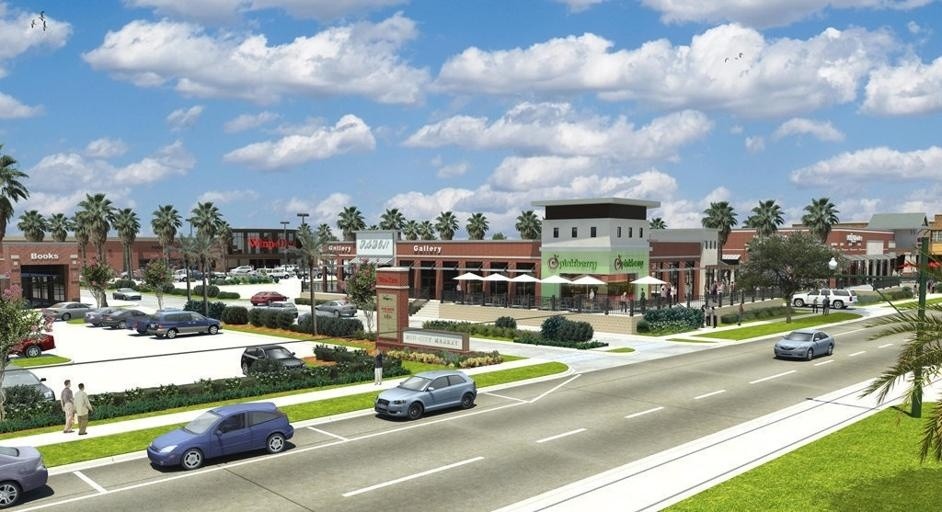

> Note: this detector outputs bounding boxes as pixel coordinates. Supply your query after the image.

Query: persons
[735,301,744,327]
[911,283,918,301]
[705,306,712,327]
[587,286,597,310]
[371,349,385,386]
[927,277,937,295]
[813,296,819,315]
[699,304,705,330]
[711,305,717,328]
[60,379,77,433]
[824,297,830,317]
[74,383,94,435]
[618,281,740,314]
[822,296,827,315]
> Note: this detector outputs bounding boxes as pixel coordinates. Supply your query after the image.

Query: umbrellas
[449,270,609,299]
[630,275,667,286]
